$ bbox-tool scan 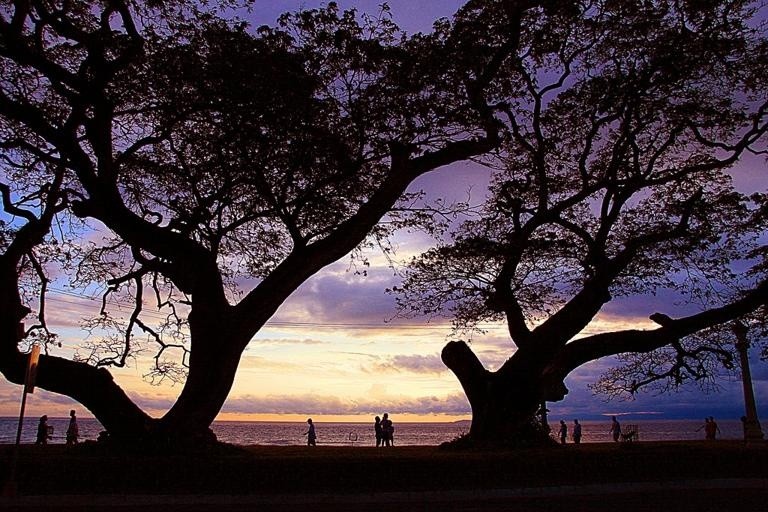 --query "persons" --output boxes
[694,417,710,440]
[707,415,720,440]
[556,420,567,443]
[41,423,52,445]
[302,419,317,446]
[571,419,581,444]
[65,410,76,445]
[740,415,749,440]
[373,412,395,446]
[33,414,49,445]
[68,417,79,445]
[607,415,621,443]
